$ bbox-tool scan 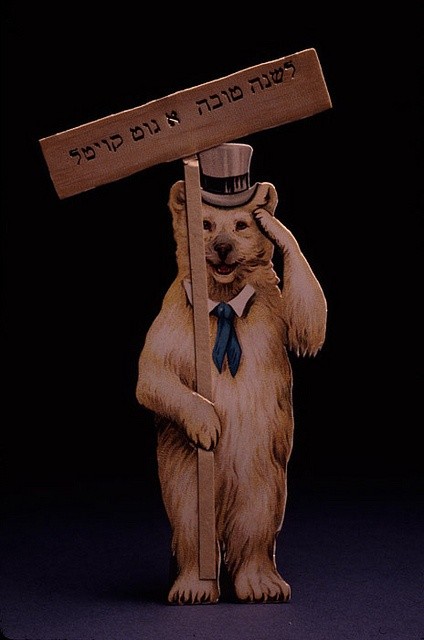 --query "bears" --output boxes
[135,175,331,606]
